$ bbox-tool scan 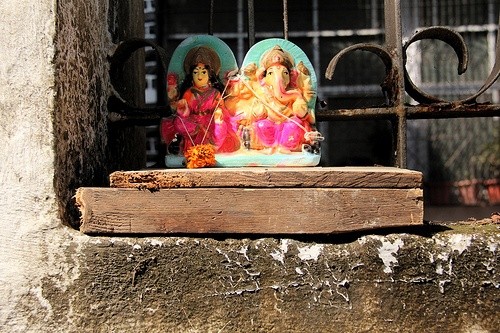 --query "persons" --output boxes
[160,45,228,157]
